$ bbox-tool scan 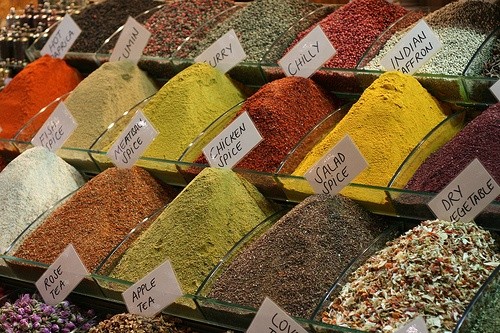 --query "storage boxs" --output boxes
[0,0,500,333]
